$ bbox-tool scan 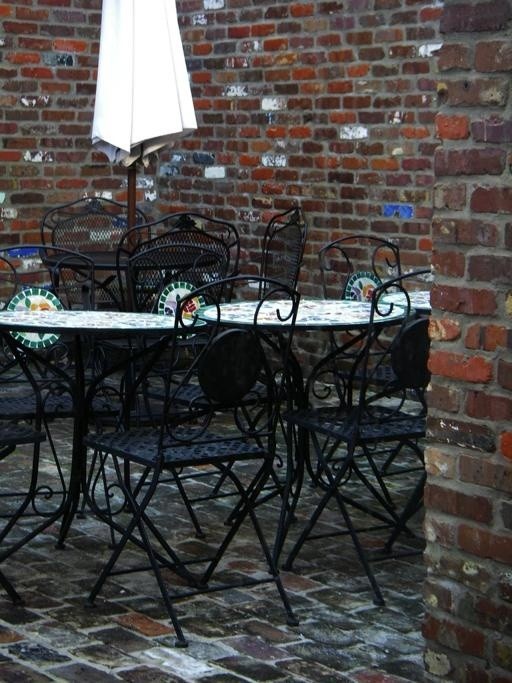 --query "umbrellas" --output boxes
[92,0,199,259]
